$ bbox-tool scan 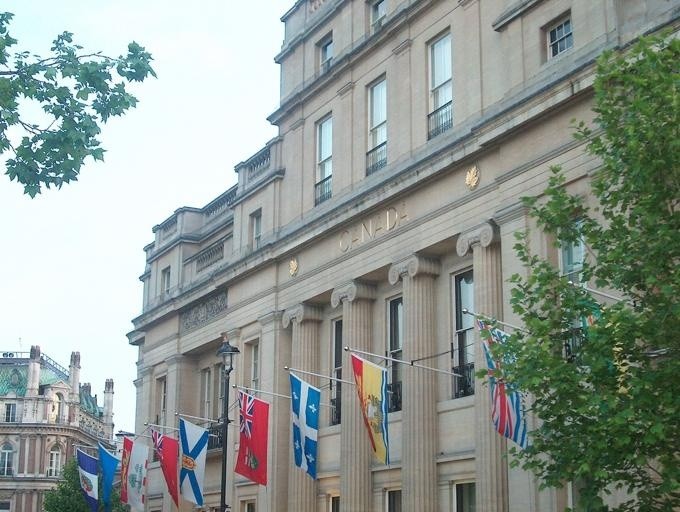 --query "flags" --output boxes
[76,449,100,512]
[234,390,269,486]
[476,319,527,450]
[565,288,602,366]
[351,352,390,467]
[179,416,210,507]
[152,427,180,511]
[98,444,120,512]
[290,372,320,481]
[121,435,150,510]
